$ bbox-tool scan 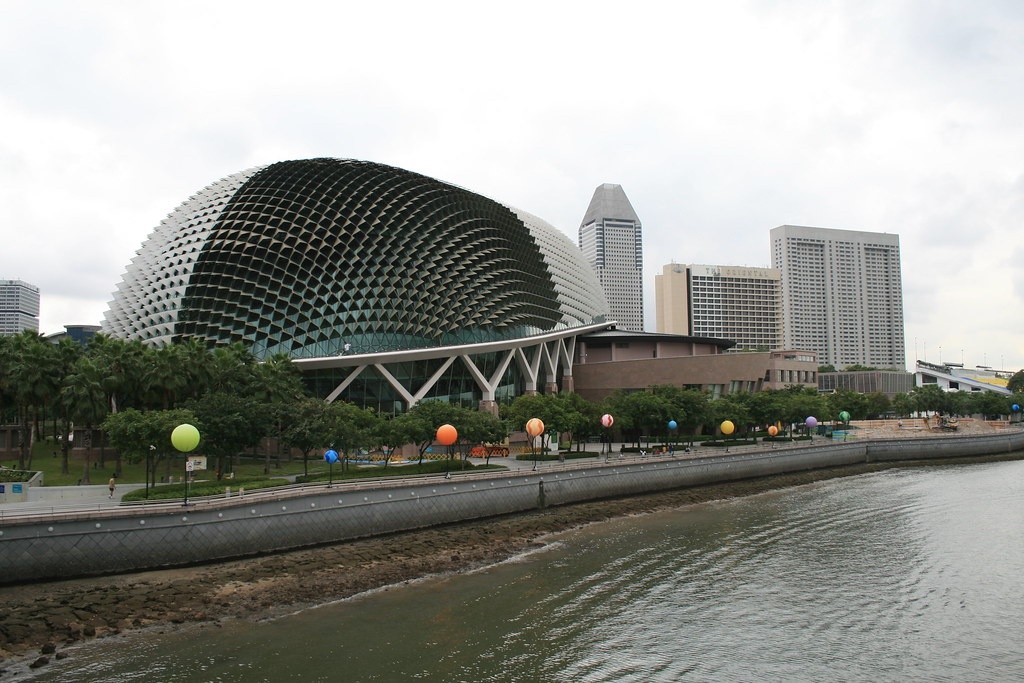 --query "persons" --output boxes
[686,446,691,454]
[651,448,660,457]
[108,474,117,499]
[897,418,903,427]
[640,449,647,458]
[619,452,625,460]
